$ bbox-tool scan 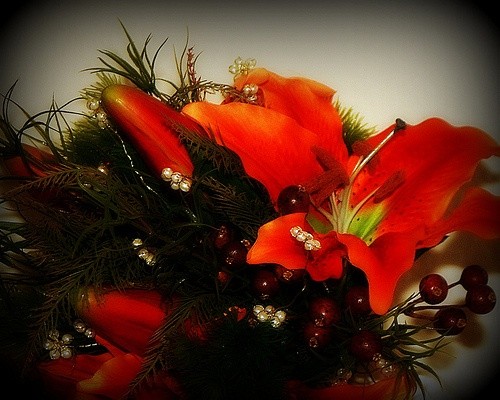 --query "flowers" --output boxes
[0,19,500,399]
